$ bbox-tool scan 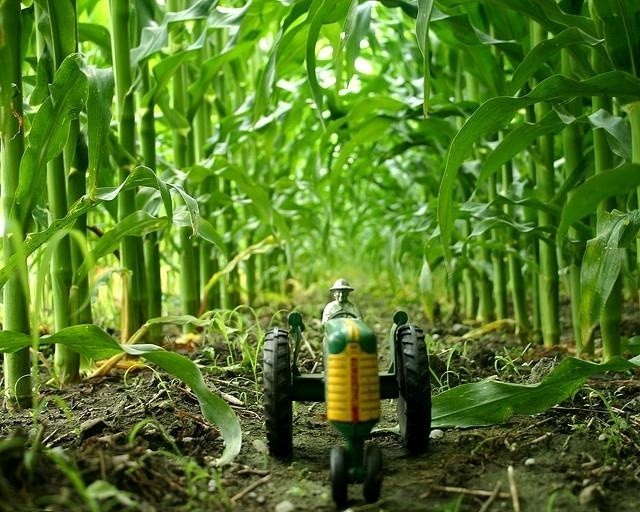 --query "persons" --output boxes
[322,279,361,325]
